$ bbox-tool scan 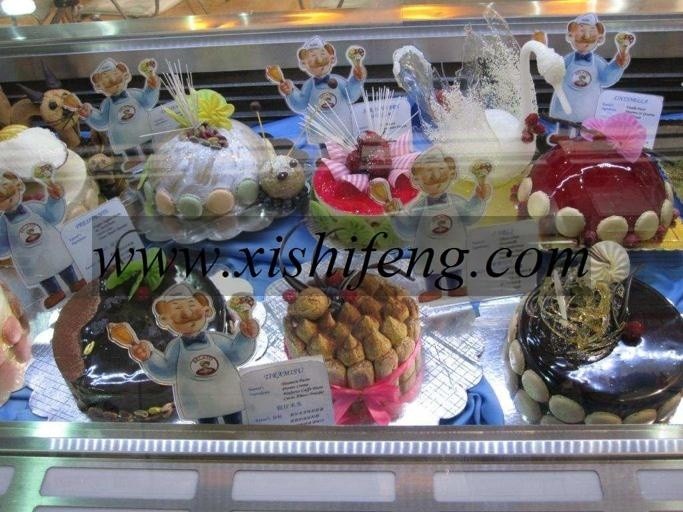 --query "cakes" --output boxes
[52,259,235,424]
[281,266,420,426]
[0,279,33,407]
[305,88,439,252]
[509,112,680,246]
[0,59,100,270]
[391,2,572,187]
[137,78,337,245]
[503,241,683,427]
